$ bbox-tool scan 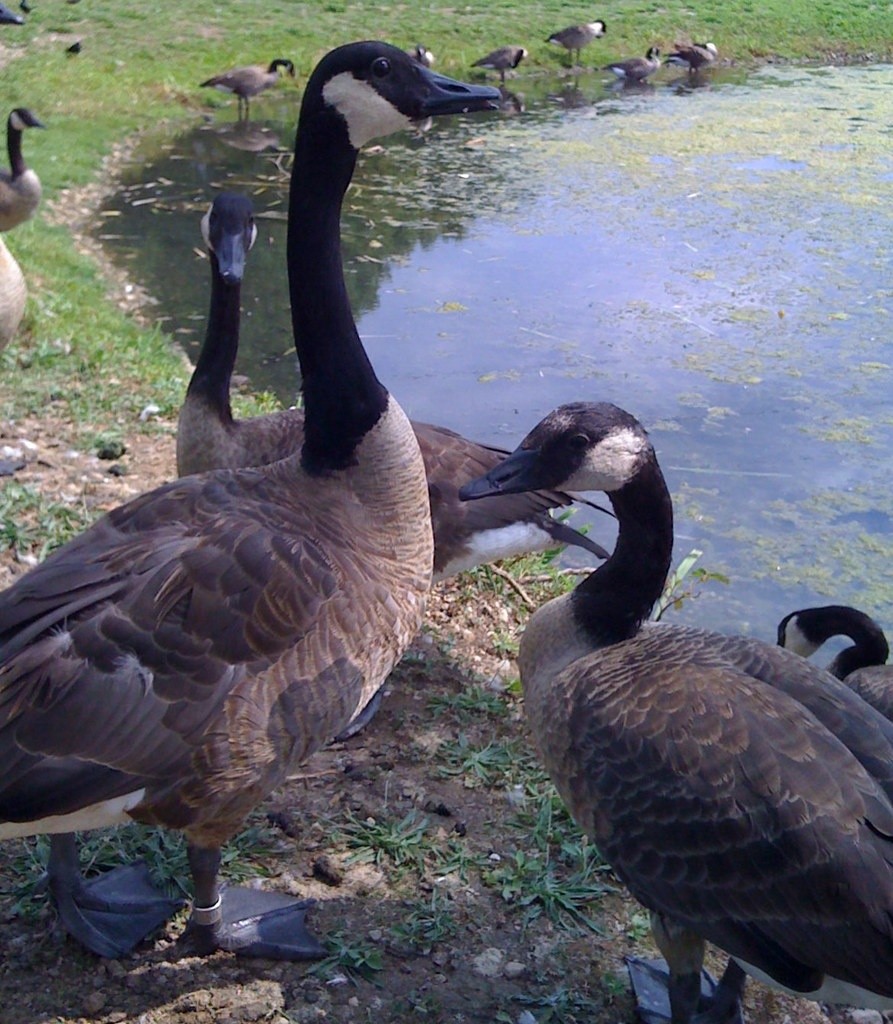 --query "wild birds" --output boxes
[0,236,28,357]
[0,108,48,233]
[200,99,290,153]
[172,187,618,587]
[775,604,893,723]
[67,43,81,54]
[406,45,435,70]
[543,18,607,56]
[469,45,528,81]
[601,48,660,81]
[0,3,25,25]
[496,84,526,118]
[0,39,503,969]
[20,0,38,14]
[197,58,295,99]
[659,39,718,74]
[459,401,893,1024]
